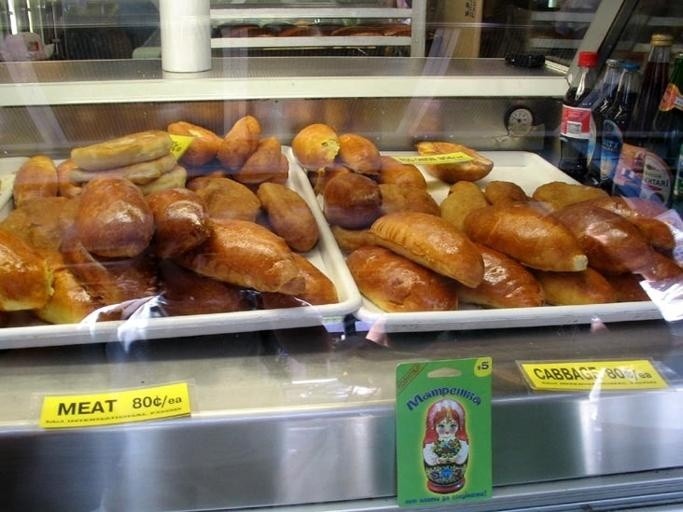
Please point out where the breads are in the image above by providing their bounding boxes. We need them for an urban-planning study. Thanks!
[291,114,682,313]
[0,115,338,326]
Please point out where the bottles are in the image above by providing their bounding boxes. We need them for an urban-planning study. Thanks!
[557,32,683,207]
